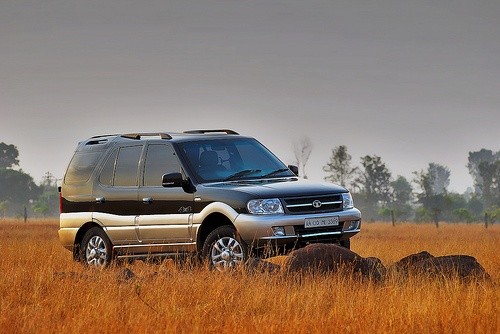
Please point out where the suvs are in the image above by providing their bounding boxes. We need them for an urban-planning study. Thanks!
[58,129,361,271]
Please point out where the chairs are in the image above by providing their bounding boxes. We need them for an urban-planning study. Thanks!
[199,151,231,179]
